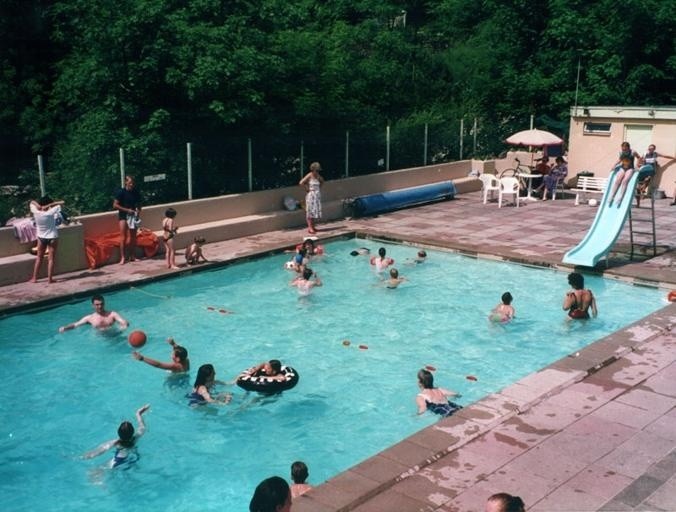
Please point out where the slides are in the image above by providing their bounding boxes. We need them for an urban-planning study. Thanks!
[562,165,654,268]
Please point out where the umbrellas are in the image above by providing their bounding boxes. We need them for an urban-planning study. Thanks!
[505,127,564,146]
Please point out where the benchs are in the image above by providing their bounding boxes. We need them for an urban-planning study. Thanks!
[570,176,609,206]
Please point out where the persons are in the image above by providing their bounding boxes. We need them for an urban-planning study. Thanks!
[527,141,676,201]
[487,493,526,512]
[162,208,180,269]
[132,335,189,392]
[30,195,65,283]
[490,292,515,322]
[416,369,464,418]
[113,175,141,265]
[249,476,293,512]
[59,295,129,335]
[290,462,312,499]
[230,359,284,419]
[185,236,208,264]
[185,364,239,417]
[284,239,426,303]
[299,161,324,233]
[562,272,597,327]
[80,403,150,471]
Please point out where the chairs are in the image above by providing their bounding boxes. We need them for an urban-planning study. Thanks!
[478,174,500,204]
[544,175,565,201]
[498,177,520,208]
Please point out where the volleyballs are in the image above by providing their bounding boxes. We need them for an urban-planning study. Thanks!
[129,331,146,347]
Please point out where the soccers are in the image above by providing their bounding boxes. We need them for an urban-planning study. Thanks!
[589,199,597,207]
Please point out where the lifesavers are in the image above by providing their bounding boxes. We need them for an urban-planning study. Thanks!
[296,244,324,255]
[370,256,393,266]
[236,365,298,392]
